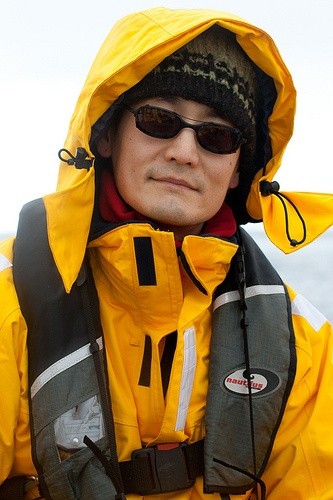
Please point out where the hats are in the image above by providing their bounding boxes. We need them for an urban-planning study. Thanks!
[124,26,261,165]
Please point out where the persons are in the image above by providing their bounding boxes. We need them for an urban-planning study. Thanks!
[0,7,333,500]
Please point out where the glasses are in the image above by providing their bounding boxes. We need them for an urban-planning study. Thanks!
[127,104,247,154]
